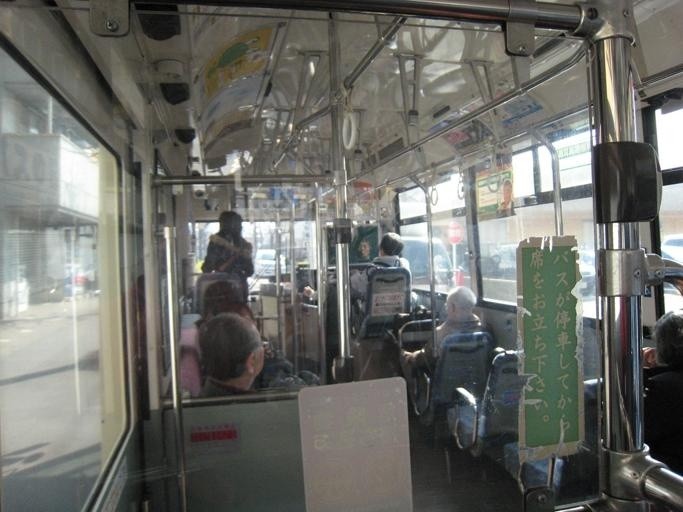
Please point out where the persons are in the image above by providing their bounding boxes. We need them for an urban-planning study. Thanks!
[195,311,265,399]
[303,285,318,306]
[203,211,254,303]
[399,286,483,380]
[209,303,256,328]
[642,310,683,474]
[202,280,242,316]
[352,238,374,263]
[500,181,512,209]
[350,231,411,321]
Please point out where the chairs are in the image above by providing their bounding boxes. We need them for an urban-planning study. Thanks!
[410,333,492,448]
[445,350,520,484]
[398,318,440,416]
[357,267,412,381]
[192,271,249,314]
[505,378,603,512]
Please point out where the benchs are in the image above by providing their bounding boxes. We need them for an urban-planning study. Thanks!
[177,311,203,397]
